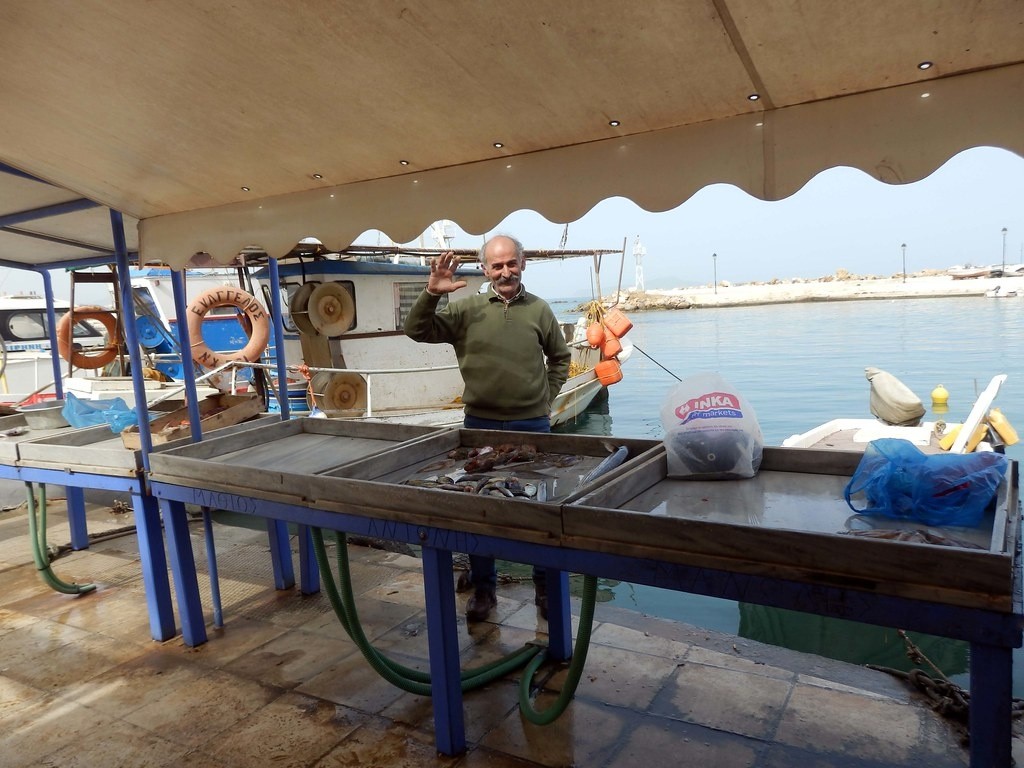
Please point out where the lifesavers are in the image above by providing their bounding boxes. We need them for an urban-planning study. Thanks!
[182,285,269,372]
[54,305,124,370]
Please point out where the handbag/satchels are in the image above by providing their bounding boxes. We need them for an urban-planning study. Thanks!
[657,370,763,477]
[844,438,1008,526]
[862,367,927,426]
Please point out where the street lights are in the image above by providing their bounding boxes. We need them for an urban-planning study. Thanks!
[901,243,907,283]
[711,253,720,295]
[1001,227,1008,275]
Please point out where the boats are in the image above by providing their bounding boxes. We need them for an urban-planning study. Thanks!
[777,366,1017,462]
[13,240,637,436]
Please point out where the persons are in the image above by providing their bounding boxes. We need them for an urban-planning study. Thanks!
[403,235,573,620]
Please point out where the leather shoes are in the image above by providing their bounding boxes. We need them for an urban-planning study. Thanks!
[466,586,498,619]
[534,590,550,622]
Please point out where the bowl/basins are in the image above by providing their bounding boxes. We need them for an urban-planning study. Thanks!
[15,398,90,430]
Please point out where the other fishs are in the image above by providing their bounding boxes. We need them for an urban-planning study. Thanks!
[399,474,536,500]
